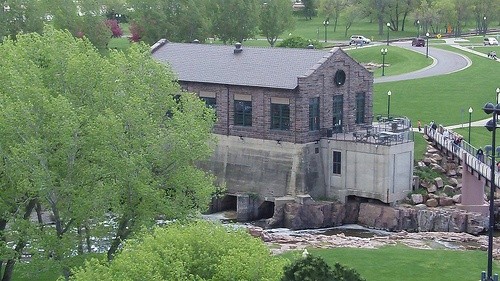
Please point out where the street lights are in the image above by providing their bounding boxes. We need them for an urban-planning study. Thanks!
[482,102,500,281]
[387,22,390,46]
[417,19,419,38]
[387,90,392,119]
[468,106,473,145]
[483,16,486,37]
[496,88,500,122]
[381,48,387,76]
[323,20,329,42]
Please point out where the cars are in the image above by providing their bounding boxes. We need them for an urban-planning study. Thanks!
[483,37,498,46]
[412,38,425,48]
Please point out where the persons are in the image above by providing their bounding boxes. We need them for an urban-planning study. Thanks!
[430,120,461,153]
[487,50,497,60]
[476,146,483,162]
[417,119,423,131]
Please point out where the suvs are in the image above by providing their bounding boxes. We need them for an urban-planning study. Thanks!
[425,32,430,58]
[350,35,371,44]
